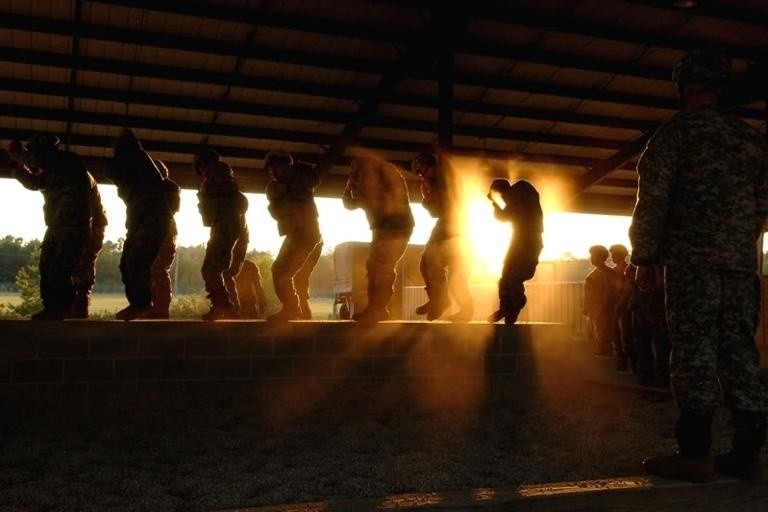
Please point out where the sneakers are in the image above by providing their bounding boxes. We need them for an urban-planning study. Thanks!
[488,292,529,325]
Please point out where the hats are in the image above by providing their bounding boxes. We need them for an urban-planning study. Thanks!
[112,127,143,155]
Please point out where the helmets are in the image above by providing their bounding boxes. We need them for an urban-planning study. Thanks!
[412,153,437,170]
[589,245,608,258]
[610,244,628,257]
[192,148,223,175]
[264,149,293,172]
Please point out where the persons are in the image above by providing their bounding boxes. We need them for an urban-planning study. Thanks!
[407,144,477,322]
[584,49,768,482]
[100,126,181,319]
[11,132,110,321]
[189,142,267,322]
[261,148,324,322]
[339,149,415,321]
[482,172,543,322]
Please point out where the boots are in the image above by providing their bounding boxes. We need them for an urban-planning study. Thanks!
[267,296,312,321]
[593,344,667,386]
[32,292,88,319]
[643,411,718,482]
[715,410,766,477]
[416,284,474,323]
[352,284,395,321]
[201,289,239,320]
[116,289,171,318]
